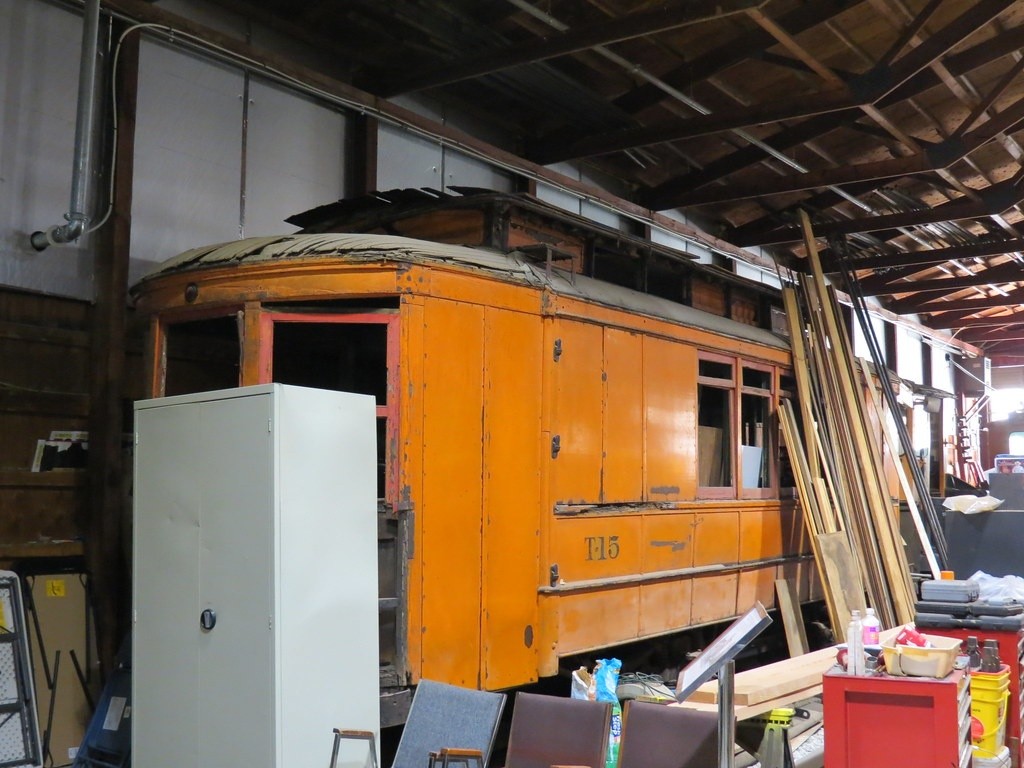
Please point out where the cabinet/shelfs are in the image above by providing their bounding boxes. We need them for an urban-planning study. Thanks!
[130,383,382,768]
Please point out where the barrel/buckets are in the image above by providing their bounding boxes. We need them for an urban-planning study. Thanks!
[969,664,1012,768]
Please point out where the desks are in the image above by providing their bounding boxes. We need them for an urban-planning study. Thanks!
[25,571,100,768]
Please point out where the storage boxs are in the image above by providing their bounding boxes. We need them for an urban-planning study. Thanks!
[880,634,963,678]
[389,203,788,335]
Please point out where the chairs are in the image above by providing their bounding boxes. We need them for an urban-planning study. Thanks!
[616,699,737,768]
[440,690,615,768]
[329,680,508,768]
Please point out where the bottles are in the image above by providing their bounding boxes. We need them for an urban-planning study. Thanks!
[862,608,880,646]
[847,610,865,676]
